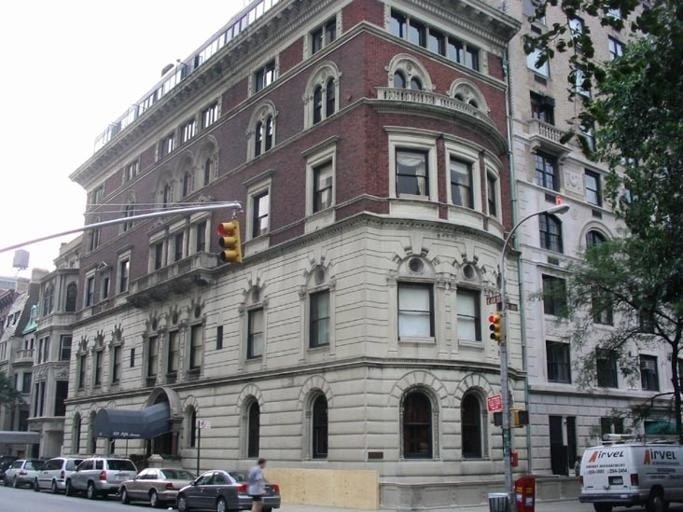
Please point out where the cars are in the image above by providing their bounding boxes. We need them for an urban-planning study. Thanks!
[0,453,283,512]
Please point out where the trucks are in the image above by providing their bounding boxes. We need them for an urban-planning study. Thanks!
[577,433,683,511]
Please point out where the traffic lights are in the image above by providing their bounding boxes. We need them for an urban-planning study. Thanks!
[487,313,503,342]
[215,218,245,268]
[508,407,528,428]
[492,410,503,426]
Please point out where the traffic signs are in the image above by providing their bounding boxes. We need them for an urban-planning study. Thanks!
[505,303,518,311]
[485,294,501,305]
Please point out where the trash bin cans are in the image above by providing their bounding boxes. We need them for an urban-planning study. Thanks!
[488,493,510,512]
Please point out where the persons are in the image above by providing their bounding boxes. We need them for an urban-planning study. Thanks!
[244,457,269,512]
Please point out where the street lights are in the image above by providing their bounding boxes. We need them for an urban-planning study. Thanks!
[496,203,570,511]
